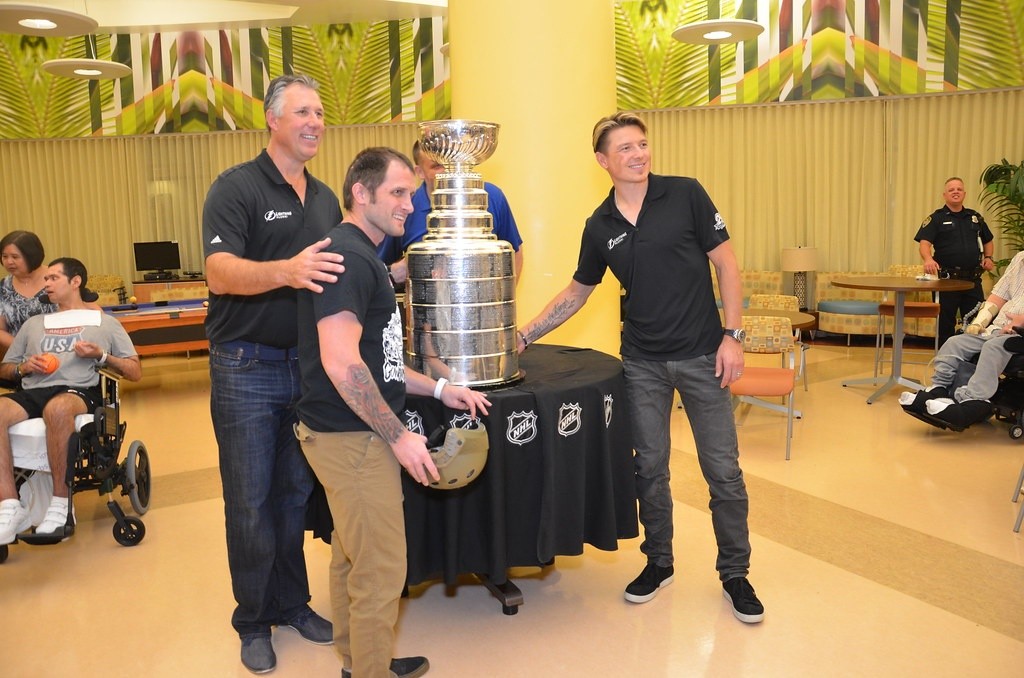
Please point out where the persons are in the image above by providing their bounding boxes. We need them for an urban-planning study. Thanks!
[294,146,493,678]
[0,258,143,545]
[899,250,1024,414]
[515,112,765,623]
[0,231,59,349]
[375,138,522,294]
[419,254,477,386]
[203,75,345,676]
[914,177,994,354]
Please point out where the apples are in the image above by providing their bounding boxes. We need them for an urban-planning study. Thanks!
[130,296,137,303]
[202,301,209,307]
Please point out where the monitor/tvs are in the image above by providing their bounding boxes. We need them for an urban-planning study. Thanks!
[134,241,181,275]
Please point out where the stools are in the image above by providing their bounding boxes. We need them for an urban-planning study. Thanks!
[819,300,880,314]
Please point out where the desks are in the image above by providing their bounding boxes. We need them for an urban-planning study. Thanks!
[676,309,816,407]
[130,280,209,302]
[303,342,639,615]
[100,296,209,359]
[830,276,975,404]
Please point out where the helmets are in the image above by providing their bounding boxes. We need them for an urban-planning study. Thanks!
[422,422,488,491]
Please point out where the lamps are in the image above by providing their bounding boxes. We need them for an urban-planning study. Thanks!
[671,18,764,46]
[782,245,817,311]
[0,0,132,83]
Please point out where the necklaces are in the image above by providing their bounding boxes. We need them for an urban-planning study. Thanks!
[22,281,31,286]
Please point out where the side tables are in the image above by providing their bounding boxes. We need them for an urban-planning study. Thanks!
[792,310,819,344]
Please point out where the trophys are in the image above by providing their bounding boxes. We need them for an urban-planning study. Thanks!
[405,120,526,389]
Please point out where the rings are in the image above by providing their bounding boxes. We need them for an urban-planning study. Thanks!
[737,373,741,376]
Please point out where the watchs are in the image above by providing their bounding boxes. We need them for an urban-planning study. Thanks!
[724,329,746,342]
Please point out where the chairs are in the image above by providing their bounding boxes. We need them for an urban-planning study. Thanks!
[874,263,942,389]
[728,295,810,461]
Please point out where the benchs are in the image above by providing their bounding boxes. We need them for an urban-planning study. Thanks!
[709,270,783,308]
[88,276,129,306]
[814,270,995,337]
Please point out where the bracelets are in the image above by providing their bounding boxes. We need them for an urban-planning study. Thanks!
[984,256,991,259]
[449,367,456,384]
[387,265,398,287]
[95,351,107,364]
[434,377,449,401]
[517,330,527,350]
[15,362,32,379]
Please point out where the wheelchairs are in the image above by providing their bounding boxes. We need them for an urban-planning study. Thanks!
[0,366,151,564]
[900,325,1024,440]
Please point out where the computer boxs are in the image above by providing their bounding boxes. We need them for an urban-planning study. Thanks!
[143,273,172,279]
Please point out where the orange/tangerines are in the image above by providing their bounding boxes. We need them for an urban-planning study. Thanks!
[38,354,59,374]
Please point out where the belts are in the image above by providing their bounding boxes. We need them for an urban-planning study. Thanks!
[210,339,298,361]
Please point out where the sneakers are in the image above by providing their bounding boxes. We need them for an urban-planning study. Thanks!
[624,563,673,604]
[0,499,32,545]
[722,576,764,623]
[240,638,276,673]
[36,496,77,535]
[341,656,430,678]
[270,607,334,645]
